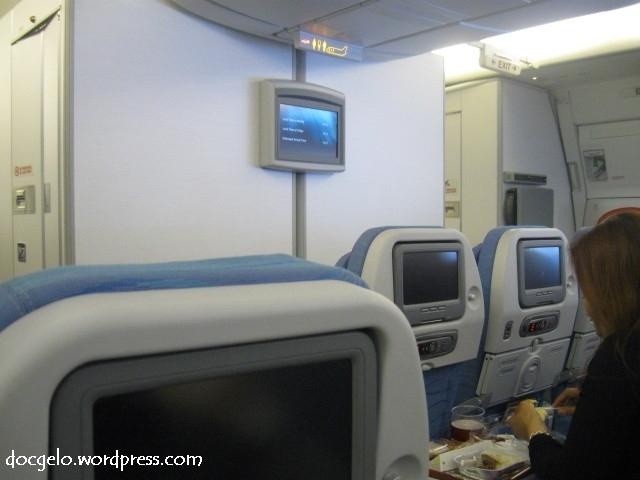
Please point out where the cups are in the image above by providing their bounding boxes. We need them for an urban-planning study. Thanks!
[450,405,487,442]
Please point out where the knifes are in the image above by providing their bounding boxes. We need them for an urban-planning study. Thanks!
[509,406,559,416]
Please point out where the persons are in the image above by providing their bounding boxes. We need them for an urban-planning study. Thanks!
[511,212,640,480]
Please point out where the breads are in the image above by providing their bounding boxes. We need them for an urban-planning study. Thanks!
[481,450,520,470]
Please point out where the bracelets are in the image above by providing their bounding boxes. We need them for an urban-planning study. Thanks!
[528,431,550,443]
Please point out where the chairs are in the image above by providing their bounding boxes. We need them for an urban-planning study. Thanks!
[0,223,606,480]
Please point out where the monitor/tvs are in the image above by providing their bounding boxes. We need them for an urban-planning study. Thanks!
[518,239,566,307]
[260,78,347,172]
[50,330,378,480]
[393,242,466,327]
